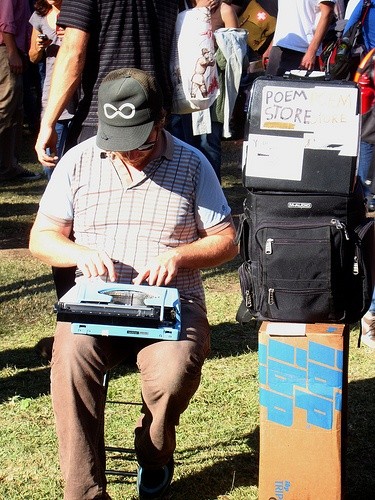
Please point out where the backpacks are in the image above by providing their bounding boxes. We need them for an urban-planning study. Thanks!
[235,175,374,325]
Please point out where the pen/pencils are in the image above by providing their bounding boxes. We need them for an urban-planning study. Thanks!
[75,258,120,277]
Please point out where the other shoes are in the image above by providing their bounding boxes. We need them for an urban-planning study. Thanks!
[361,311,375,348]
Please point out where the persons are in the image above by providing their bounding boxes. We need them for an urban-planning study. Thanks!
[29,70,239,500]
[0,0,375,357]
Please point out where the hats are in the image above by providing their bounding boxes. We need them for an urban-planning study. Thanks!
[96,68,162,152]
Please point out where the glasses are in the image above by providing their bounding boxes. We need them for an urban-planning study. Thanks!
[134,127,159,151]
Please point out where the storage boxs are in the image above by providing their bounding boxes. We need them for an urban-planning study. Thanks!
[257,321,349,500]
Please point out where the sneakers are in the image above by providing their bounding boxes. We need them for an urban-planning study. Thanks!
[137,455,174,500]
[19,169,41,180]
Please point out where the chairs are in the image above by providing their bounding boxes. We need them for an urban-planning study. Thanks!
[51,233,143,479]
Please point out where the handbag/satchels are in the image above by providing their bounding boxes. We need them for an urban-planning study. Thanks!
[320,0,371,80]
[239,0,277,51]
[170,0,220,114]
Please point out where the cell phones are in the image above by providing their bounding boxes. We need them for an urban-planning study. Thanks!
[38,34,48,40]
